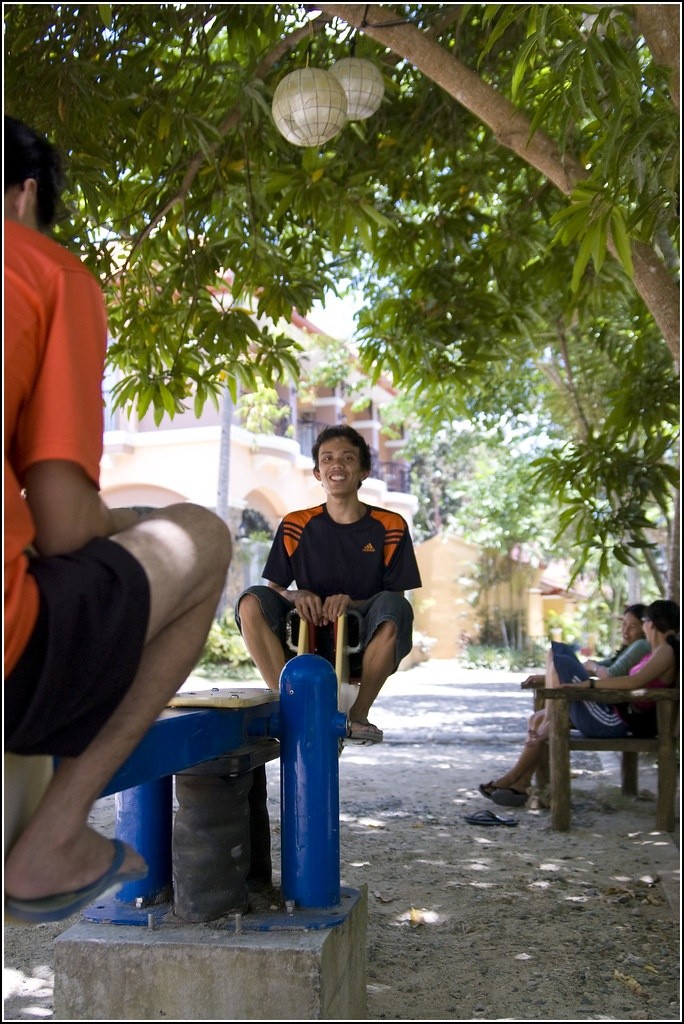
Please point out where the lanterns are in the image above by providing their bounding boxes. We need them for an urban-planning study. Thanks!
[328,58,384,121]
[272,66,348,147]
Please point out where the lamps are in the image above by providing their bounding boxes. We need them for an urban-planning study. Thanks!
[271,14,347,147]
[328,36,386,122]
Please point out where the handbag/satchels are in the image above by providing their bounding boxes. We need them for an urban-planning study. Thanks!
[616,702,658,738]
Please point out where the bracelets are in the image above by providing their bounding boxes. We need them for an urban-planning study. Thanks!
[594,666,599,672]
[590,679,594,688]
[132,506,153,517]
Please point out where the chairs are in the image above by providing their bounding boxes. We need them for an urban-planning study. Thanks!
[520,678,679,830]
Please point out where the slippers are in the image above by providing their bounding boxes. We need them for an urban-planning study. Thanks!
[464,810,520,827]
[479,781,529,807]
[4,839,148,922]
[349,720,383,739]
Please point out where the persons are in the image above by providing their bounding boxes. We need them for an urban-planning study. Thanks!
[522,604,649,742]
[235,425,422,743]
[5,113,232,924]
[479,598,680,808]
